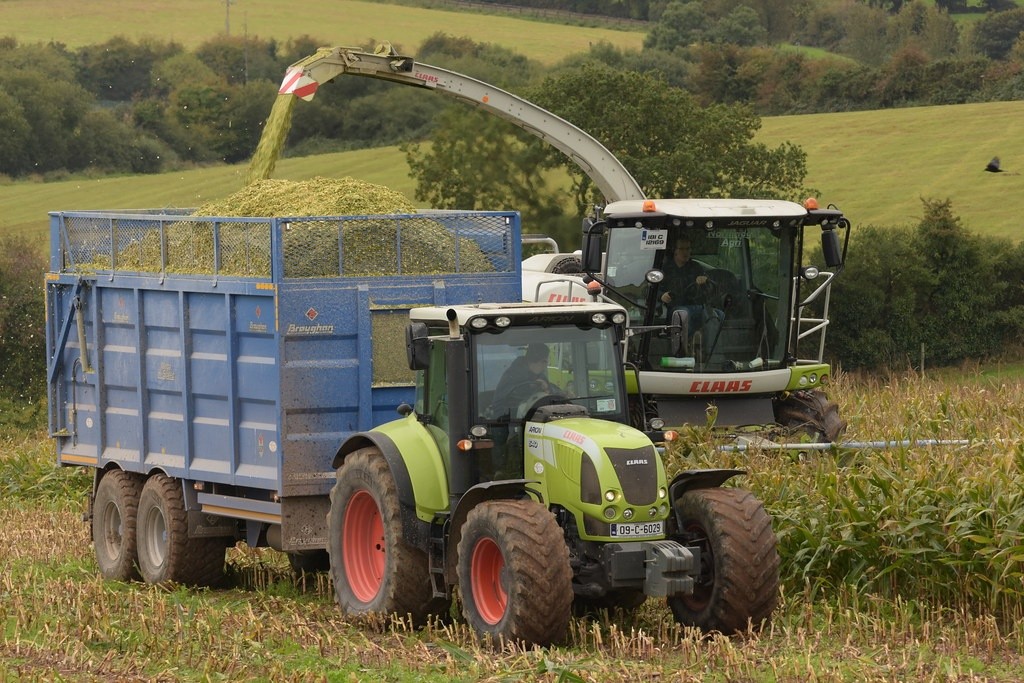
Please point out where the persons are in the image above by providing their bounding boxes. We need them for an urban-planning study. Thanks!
[641,235,709,315]
[493,343,550,410]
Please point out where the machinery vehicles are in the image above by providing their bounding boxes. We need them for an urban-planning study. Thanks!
[243,42,854,458]
[42,209,781,644]
